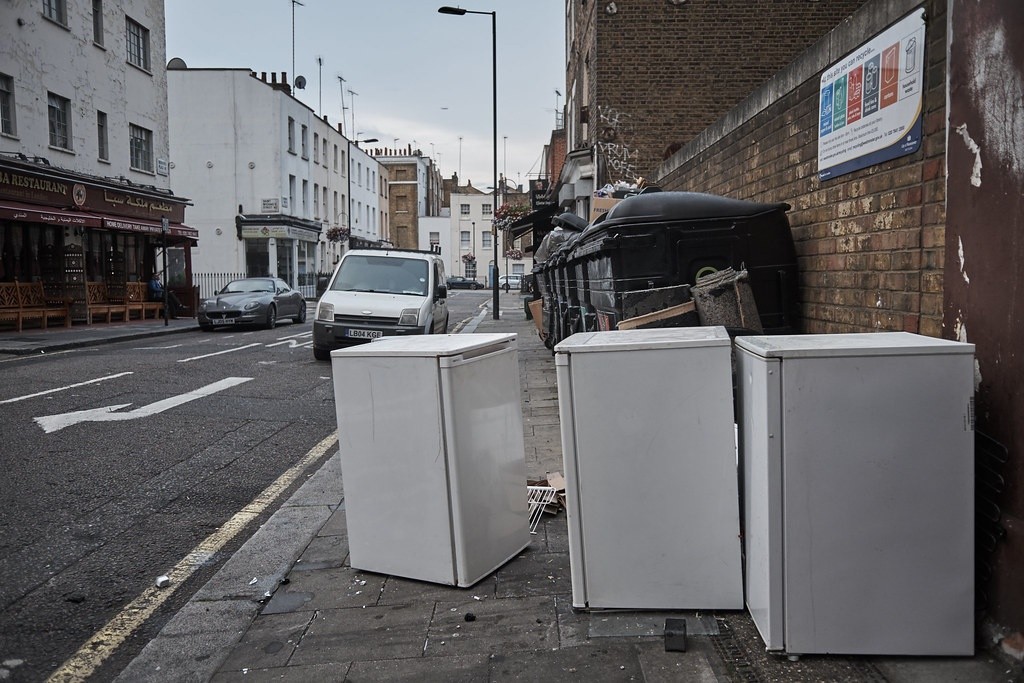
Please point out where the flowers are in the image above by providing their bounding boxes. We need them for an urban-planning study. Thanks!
[326,225,350,244]
[505,248,526,260]
[493,200,531,231]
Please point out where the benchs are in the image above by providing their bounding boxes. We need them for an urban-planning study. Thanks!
[1,281,166,337]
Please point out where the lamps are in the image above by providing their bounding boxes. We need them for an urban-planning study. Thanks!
[26,156,50,166]
[146,186,174,196]
[105,176,132,186]
[125,183,156,192]
[0,150,28,161]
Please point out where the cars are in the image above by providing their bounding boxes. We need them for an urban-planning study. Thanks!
[446,276,484,290]
[198,278,306,332]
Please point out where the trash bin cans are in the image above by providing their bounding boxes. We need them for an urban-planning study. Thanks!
[529,190,802,356]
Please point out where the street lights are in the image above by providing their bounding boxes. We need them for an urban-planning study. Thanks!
[347,138,378,249]
[437,5,500,319]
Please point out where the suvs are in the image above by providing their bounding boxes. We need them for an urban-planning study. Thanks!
[499,275,524,290]
[310,236,451,361]
